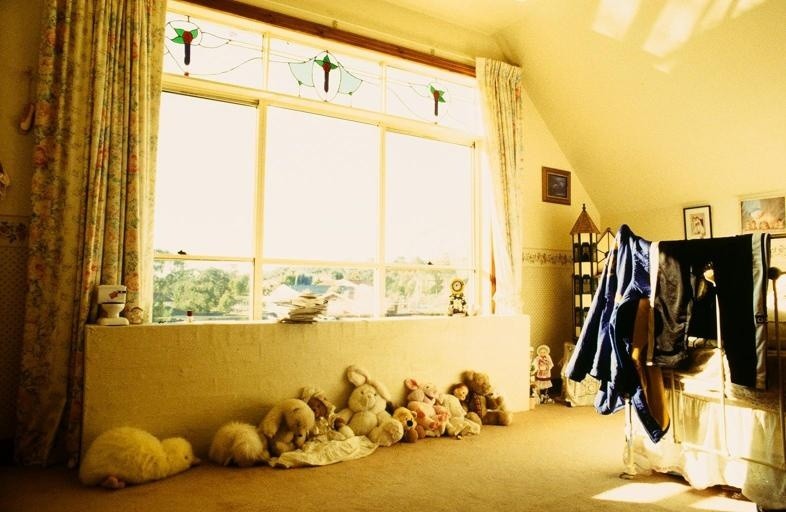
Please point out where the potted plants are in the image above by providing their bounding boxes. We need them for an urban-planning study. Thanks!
[736,193,786,235]
[683,205,713,240]
[542,166,571,206]
